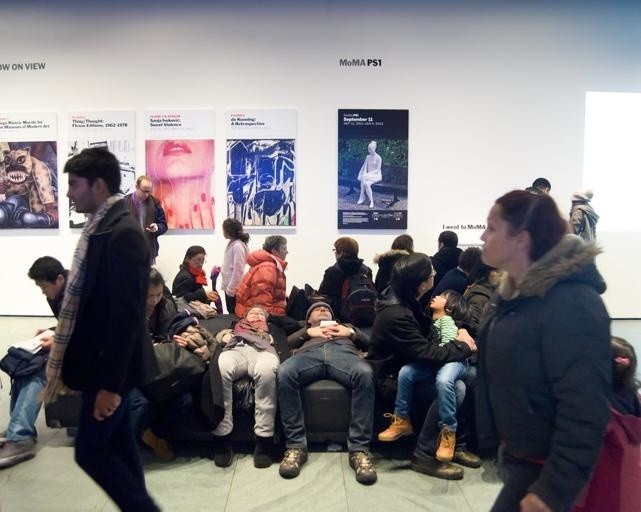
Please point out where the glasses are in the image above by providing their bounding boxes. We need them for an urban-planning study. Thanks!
[429,269,436,277]
[138,186,149,193]
[510,187,543,235]
[332,249,336,253]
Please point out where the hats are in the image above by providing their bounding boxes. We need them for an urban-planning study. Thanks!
[305,302,333,325]
[571,190,593,202]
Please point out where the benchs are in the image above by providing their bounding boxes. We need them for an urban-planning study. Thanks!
[44,314,474,448]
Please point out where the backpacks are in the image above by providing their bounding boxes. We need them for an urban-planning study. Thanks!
[334,263,378,329]
[568,407,641,512]
[0,347,48,395]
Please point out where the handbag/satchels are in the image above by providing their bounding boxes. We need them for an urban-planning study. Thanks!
[147,336,206,392]
[304,284,331,306]
[287,286,307,320]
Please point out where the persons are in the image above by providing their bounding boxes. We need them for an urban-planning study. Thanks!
[479,189,610,511]
[122,176,168,265]
[145,141,214,232]
[356,140,382,208]
[525,178,551,196]
[569,189,601,244]
[62,146,162,512]
[136,219,501,485]
[0,256,70,468]
[570,336,641,512]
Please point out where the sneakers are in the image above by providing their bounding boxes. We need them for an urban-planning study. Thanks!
[0,438,35,466]
[278,448,306,477]
[141,427,175,460]
[376,413,413,442]
[0,425,37,442]
[211,434,233,466]
[348,449,376,485]
[411,426,482,480]
[253,436,273,468]
